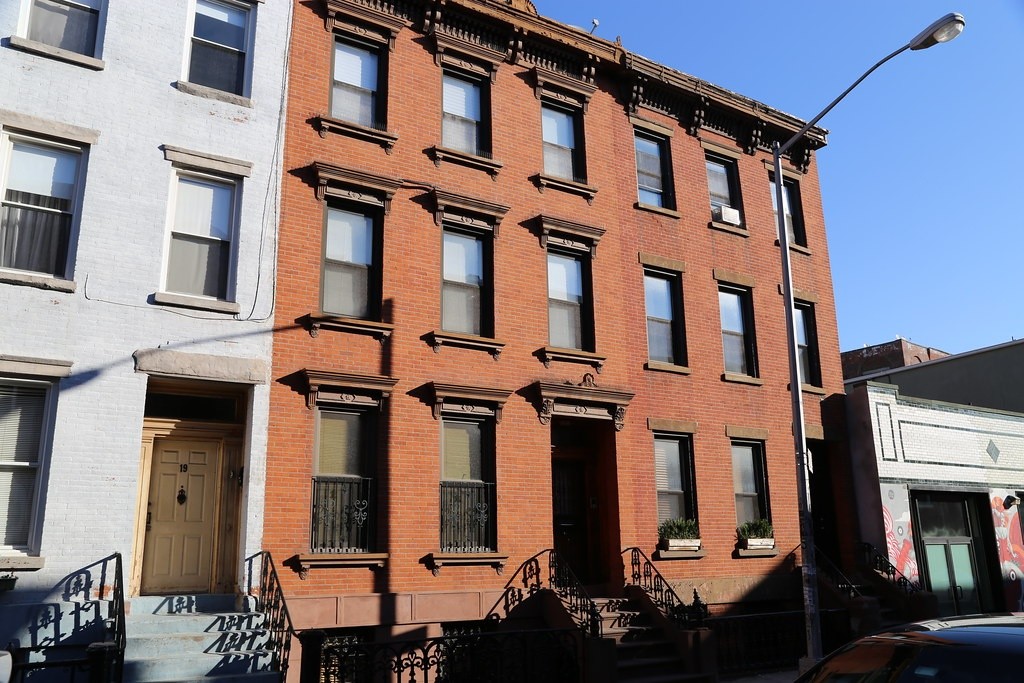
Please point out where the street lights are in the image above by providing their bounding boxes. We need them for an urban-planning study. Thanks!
[773,13,967,670]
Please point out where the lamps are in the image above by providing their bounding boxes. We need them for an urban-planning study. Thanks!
[1005,494,1021,506]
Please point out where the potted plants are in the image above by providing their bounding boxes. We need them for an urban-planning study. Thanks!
[736,518,775,550]
[0,572,19,590]
[658,515,701,550]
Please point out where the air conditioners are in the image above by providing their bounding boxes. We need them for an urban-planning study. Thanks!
[714,206,740,227]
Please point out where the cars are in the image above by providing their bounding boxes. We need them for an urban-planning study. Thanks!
[793,613,1024,683]
